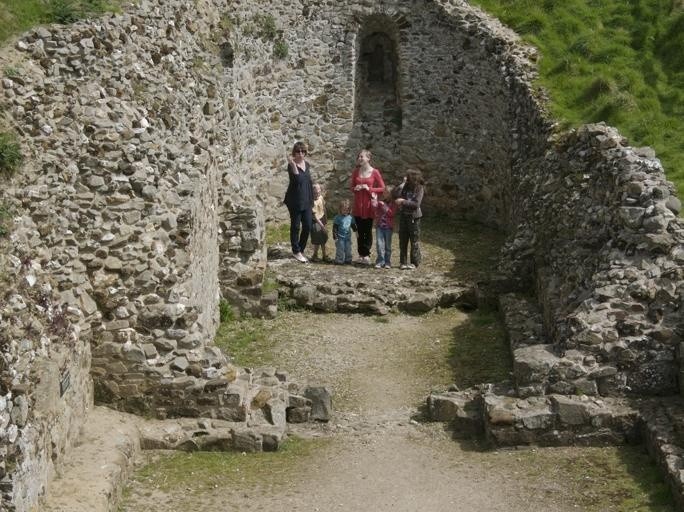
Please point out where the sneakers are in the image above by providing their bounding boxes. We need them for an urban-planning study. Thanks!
[291,252,418,269]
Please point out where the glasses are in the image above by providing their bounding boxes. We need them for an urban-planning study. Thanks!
[295,150,306,154]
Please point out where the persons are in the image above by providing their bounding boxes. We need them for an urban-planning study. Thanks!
[349,148,386,264]
[392,167,424,269]
[330,199,359,265]
[281,141,314,264]
[309,182,333,263]
[369,184,398,270]
